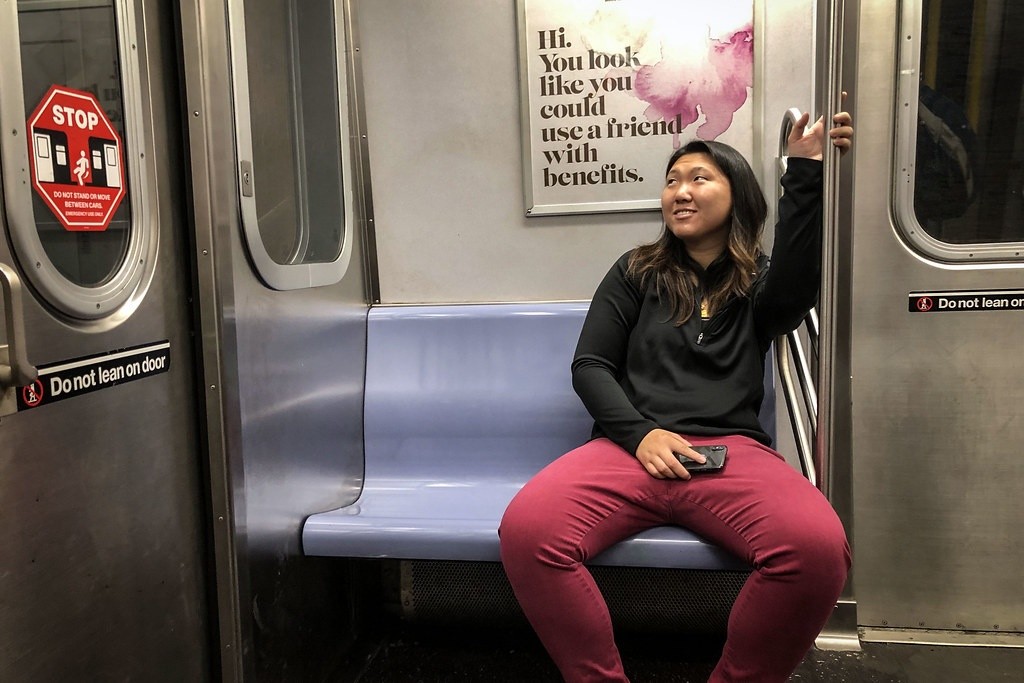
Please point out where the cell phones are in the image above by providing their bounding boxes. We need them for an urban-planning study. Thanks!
[671,445,729,471]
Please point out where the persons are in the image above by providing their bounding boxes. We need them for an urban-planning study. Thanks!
[499,91,856,683]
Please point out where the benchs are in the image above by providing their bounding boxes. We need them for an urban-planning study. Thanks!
[301,296,784,636]
